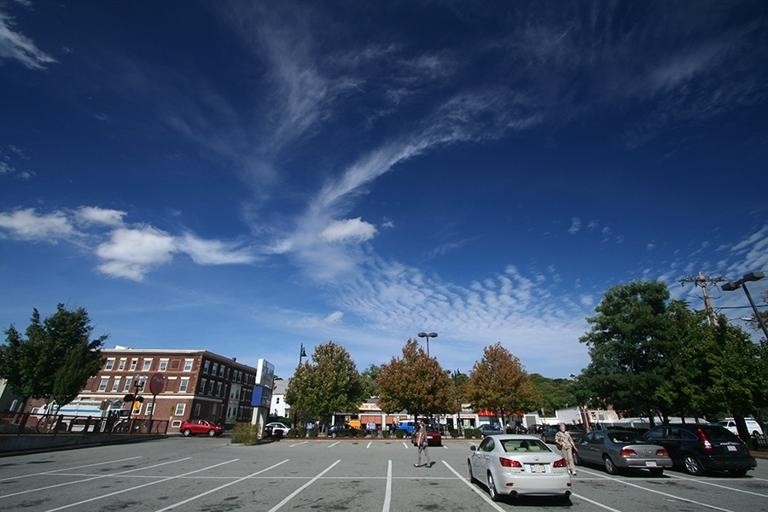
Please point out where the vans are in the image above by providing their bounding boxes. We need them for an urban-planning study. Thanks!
[721,420,764,438]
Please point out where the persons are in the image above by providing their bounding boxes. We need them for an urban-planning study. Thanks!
[413,421,432,469]
[554,422,580,476]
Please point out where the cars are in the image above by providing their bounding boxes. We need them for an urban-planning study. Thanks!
[642,423,757,476]
[328,424,366,438]
[572,428,674,477]
[264,421,292,437]
[470,423,551,434]
[180,417,224,437]
[467,433,572,502]
[393,421,418,439]
[411,424,441,446]
[542,424,586,443]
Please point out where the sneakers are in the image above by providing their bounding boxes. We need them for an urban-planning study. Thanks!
[414,463,431,468]
[568,470,577,475]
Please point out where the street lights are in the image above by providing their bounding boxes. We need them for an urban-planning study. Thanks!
[721,271,768,339]
[418,331,438,359]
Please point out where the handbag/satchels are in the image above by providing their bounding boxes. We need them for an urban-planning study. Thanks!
[414,437,424,447]
[556,442,562,450]
